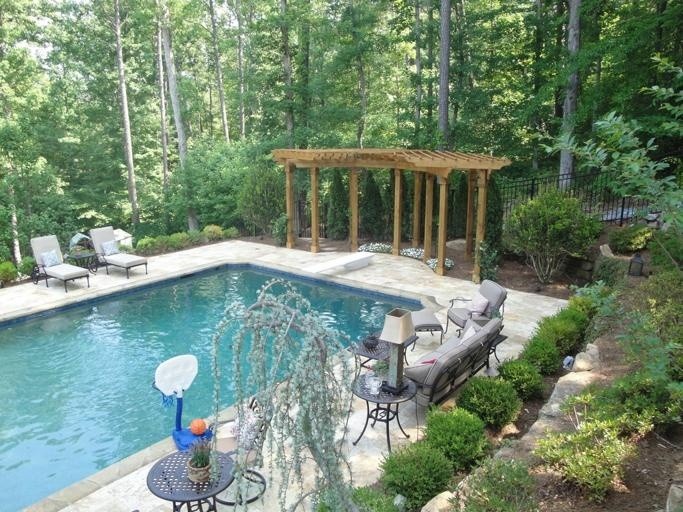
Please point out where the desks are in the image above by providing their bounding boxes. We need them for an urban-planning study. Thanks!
[488,335,508,365]
[348,369,419,459]
[144,447,240,512]
[66,252,97,276]
[347,330,420,377]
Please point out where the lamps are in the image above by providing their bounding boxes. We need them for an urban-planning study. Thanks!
[370,306,423,397]
[625,248,646,279]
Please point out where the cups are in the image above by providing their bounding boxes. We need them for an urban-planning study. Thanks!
[363,371,381,396]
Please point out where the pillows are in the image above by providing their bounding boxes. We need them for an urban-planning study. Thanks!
[37,248,64,271]
[100,239,123,259]
[456,324,477,346]
[464,290,491,318]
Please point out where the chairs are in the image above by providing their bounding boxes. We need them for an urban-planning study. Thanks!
[28,234,91,294]
[89,225,148,282]
[205,391,276,506]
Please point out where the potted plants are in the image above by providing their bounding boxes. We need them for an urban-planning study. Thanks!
[186,436,217,488]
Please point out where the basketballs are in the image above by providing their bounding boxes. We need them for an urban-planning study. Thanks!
[191,419,206,435]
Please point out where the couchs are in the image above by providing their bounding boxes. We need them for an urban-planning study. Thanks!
[409,308,446,353]
[441,278,509,339]
[406,315,509,416]
